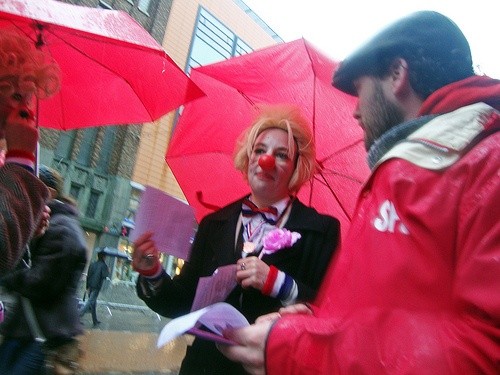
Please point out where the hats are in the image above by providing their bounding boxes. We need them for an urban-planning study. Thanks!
[331,10,472,96]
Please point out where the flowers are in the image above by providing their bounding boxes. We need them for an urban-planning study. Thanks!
[260,228,302,255]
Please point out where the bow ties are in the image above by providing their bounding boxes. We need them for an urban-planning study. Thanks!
[241,199,278,225]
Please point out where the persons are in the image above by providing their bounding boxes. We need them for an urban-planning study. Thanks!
[0,30,51,374]
[77,250,111,327]
[131,100,342,375]
[0,164,91,375]
[214,9,499,375]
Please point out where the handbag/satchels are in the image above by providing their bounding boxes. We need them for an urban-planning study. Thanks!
[39,334,86,369]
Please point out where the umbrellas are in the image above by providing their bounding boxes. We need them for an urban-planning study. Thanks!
[164,35,379,237]
[95,245,129,259]
[0,0,209,131]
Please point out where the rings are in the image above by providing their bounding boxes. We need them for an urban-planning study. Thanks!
[18,109,29,119]
[241,263,246,271]
[145,254,157,261]
